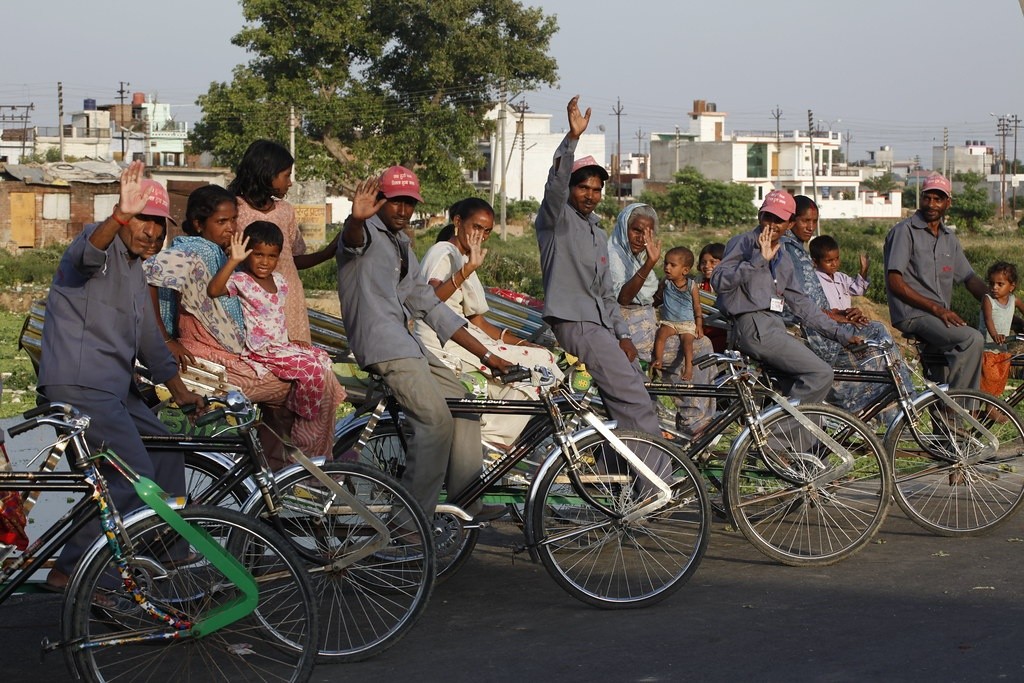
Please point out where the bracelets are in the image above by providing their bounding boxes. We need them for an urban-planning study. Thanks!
[451,273,459,289]
[462,265,469,280]
[500,327,508,340]
[480,351,493,365]
[113,211,129,226]
[515,339,526,346]
[165,338,171,343]
[636,272,646,280]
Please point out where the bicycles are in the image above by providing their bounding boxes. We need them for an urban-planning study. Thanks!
[0,335,1024,683]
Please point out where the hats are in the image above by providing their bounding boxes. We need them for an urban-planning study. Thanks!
[140,179,178,227]
[378,167,424,202]
[921,175,951,198]
[760,190,796,221]
[571,155,609,181]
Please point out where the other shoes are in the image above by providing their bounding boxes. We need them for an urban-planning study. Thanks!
[462,504,513,525]
[385,517,424,550]
[671,475,694,493]
[692,419,732,433]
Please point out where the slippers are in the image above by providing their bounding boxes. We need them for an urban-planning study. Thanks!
[763,446,792,469]
[933,410,965,436]
[154,553,204,570]
[950,467,1000,486]
[39,581,115,607]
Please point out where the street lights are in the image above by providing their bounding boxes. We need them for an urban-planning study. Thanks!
[990,112,1011,159]
[818,119,842,131]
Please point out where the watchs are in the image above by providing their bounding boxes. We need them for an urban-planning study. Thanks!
[621,334,632,340]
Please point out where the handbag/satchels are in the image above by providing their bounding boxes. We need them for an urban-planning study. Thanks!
[0,444,29,550]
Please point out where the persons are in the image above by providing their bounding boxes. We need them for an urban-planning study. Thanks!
[882,175,1024,487]
[977,261,1024,423]
[693,243,728,359]
[607,203,734,435]
[782,195,917,425]
[652,246,704,380]
[336,166,513,547]
[207,220,331,437]
[535,95,672,500]
[227,139,341,344]
[37,159,210,608]
[142,184,347,486]
[413,197,566,445]
[710,190,863,467]
[809,235,870,311]
